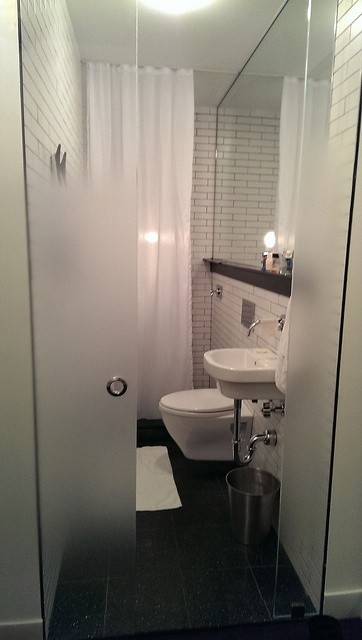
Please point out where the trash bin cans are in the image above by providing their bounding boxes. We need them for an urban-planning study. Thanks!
[225,468,280,546]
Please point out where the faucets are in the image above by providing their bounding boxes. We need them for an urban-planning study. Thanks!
[248,319,287,337]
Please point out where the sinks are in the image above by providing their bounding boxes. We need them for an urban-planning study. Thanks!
[203,347,279,402]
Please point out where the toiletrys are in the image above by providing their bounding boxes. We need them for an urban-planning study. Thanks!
[284,250,293,274]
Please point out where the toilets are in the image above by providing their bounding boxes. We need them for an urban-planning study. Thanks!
[158,389,252,461]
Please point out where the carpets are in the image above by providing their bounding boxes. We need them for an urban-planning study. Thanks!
[137,446,181,510]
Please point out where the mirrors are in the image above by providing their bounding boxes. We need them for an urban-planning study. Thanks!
[211,0,338,274]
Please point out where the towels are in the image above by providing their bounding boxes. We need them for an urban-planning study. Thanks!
[274,294,292,393]
